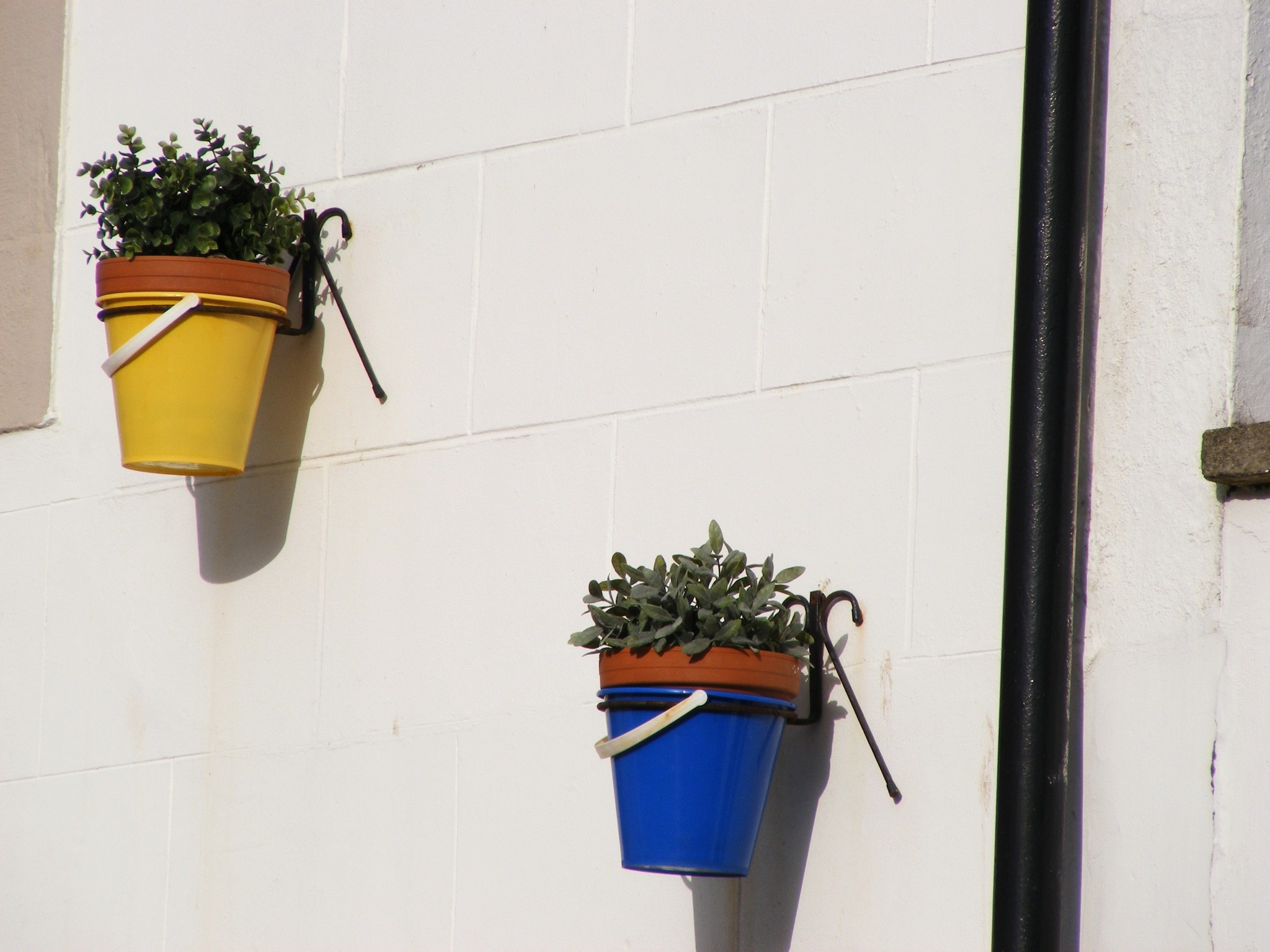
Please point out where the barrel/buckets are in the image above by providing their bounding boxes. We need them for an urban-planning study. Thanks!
[593,687,798,875]
[96,290,290,478]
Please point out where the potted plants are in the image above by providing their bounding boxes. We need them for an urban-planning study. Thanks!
[565,530,817,695]
[75,112,308,296]
